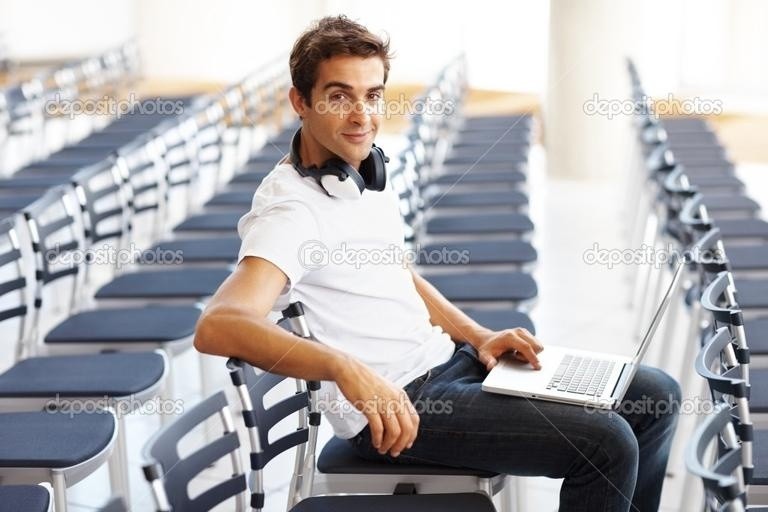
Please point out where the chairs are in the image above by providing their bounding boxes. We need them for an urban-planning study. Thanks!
[140,388,247,512]
[0,410,126,512]
[0,213,167,512]
[24,180,216,469]
[282,300,511,512]
[225,316,497,512]
[0,481,55,512]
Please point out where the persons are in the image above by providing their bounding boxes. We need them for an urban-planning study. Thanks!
[193,16,681,512]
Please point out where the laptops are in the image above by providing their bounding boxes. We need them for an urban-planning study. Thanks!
[481,257,684,411]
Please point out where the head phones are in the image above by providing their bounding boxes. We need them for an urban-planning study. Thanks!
[290,126,390,202]
